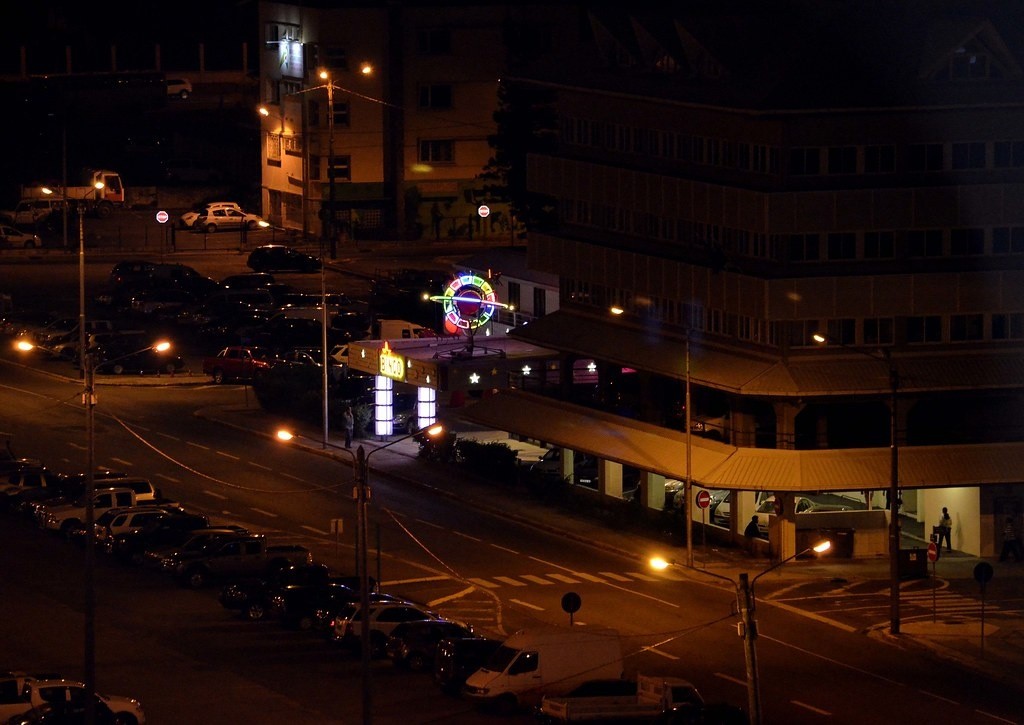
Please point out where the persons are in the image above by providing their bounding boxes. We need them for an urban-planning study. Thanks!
[861,490,873,510]
[343,407,354,449]
[744,515,768,540]
[938,507,952,553]
[883,490,901,510]
[997,517,1021,563]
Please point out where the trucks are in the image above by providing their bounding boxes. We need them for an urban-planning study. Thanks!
[20,169,125,220]
[540,672,706,725]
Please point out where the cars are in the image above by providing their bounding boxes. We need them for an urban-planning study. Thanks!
[196,206,261,233]
[0,257,1022,725]
[685,704,750,725]
[0,225,42,250]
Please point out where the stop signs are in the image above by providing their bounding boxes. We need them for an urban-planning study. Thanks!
[156,210,169,223]
[696,490,710,508]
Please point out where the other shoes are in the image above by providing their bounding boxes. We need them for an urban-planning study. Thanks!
[345,446,352,449]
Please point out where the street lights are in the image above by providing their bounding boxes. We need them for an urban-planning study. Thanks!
[252,65,396,257]
[649,538,831,725]
[262,263,329,450]
[607,304,692,569]
[811,332,899,635]
[38,178,105,434]
[273,423,444,725]
[10,335,175,725]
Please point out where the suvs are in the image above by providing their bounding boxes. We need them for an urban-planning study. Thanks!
[247,245,323,274]
[0,198,73,235]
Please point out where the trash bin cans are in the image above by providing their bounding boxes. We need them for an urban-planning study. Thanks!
[899,548,928,577]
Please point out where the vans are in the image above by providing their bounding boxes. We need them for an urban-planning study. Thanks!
[464,622,627,717]
[194,201,241,218]
